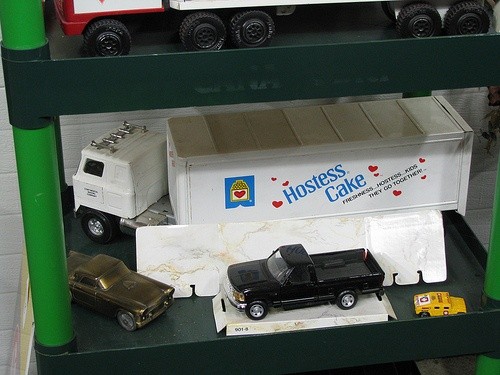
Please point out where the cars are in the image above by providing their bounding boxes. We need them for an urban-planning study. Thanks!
[66,249,175,332]
[413,291,468,319]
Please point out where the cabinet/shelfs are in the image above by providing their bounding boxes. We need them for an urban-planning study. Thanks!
[0,0,500,375]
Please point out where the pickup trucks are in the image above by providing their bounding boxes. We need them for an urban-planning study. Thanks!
[224,243,386,322]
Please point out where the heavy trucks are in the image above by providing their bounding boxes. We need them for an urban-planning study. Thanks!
[72,94,475,246]
[52,0,495,61]
